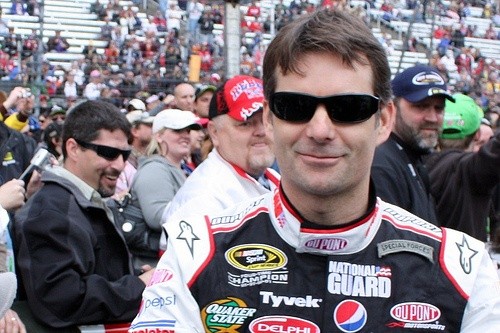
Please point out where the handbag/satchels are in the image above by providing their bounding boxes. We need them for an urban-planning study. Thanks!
[107,159,177,257]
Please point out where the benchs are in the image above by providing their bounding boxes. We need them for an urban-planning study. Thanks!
[0,0,500,91]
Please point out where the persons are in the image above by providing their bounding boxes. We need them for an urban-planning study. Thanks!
[129,7,500,333]
[0,0,500,280]
[160,77,281,257]
[373,66,455,225]
[12,100,155,333]
[0,206,26,333]
[425,93,500,243]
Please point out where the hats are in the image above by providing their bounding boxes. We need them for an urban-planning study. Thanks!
[152,110,201,132]
[130,99,147,111]
[391,64,457,103]
[441,92,483,138]
[127,111,155,123]
[48,104,65,116]
[90,70,101,77]
[208,75,264,121]
[195,84,217,97]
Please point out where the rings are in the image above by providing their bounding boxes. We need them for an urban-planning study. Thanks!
[11,317,16,320]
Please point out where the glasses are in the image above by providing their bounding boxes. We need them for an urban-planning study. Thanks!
[75,139,132,162]
[52,117,66,121]
[269,92,385,123]
[39,120,44,123]
